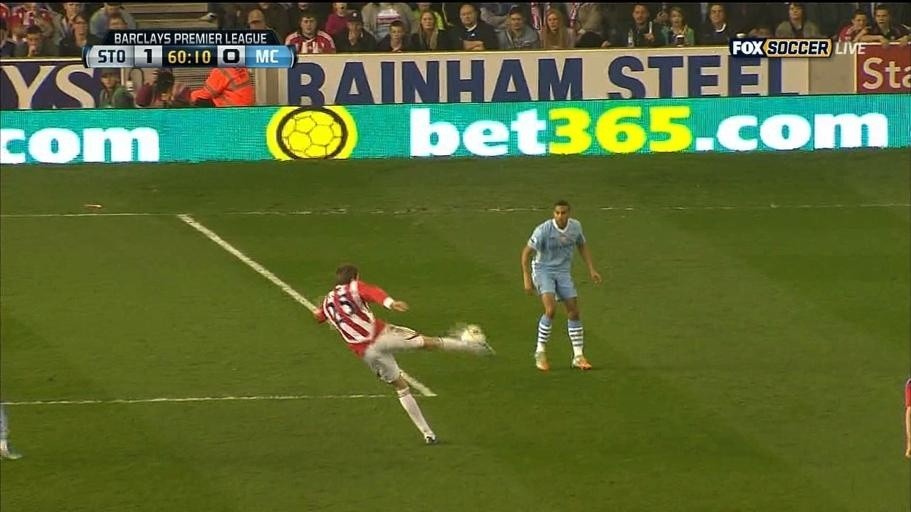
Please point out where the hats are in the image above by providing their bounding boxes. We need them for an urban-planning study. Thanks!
[346,10,363,23]
[246,9,266,26]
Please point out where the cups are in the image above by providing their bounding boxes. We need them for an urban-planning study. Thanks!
[677,34,686,45]
[736,33,748,38]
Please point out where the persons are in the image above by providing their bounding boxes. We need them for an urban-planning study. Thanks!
[1,2,910,110]
[520,199,602,371]
[312,265,496,446]
[905,377,911,459]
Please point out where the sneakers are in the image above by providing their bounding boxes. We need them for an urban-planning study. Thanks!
[0,448,25,463]
[461,328,497,358]
[424,432,439,444]
[533,350,550,370]
[569,357,594,370]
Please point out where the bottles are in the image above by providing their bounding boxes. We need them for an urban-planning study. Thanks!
[628,27,635,48]
